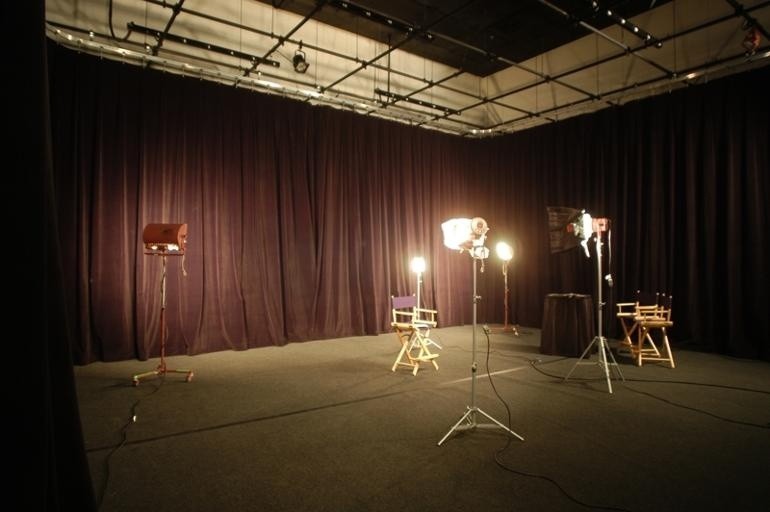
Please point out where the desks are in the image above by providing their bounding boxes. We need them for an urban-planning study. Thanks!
[538,293,598,359]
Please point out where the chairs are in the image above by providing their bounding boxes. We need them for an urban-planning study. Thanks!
[390,294,439,375]
[612,289,676,369]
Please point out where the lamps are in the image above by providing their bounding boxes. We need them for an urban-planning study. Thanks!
[741,21,762,50]
[126,222,195,257]
[292,41,309,73]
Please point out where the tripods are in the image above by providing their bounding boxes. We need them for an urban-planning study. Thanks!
[437,253,525,446]
[561,226,625,394]
